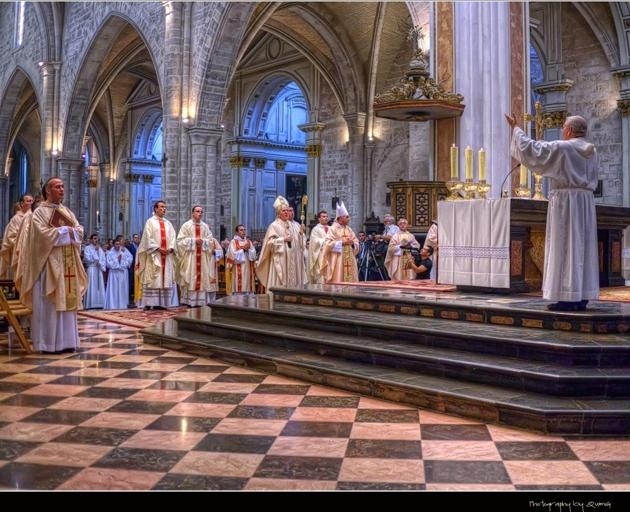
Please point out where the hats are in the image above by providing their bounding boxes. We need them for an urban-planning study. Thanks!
[384,216,393,224]
[273,196,289,210]
[336,201,349,221]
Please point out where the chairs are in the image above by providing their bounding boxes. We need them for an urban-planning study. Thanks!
[0,284,34,354]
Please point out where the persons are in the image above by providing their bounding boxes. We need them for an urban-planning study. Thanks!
[1,176,437,355]
[505,111,599,312]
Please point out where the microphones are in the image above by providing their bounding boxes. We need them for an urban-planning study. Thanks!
[501,164,521,197]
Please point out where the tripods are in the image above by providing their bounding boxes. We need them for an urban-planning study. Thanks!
[358,240,385,282]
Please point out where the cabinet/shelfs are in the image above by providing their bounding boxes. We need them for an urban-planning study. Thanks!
[387,180,413,229]
[411,181,436,230]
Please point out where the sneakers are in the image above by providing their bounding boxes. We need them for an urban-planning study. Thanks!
[548,304,577,311]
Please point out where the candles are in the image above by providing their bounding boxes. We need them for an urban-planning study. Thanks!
[95,209,100,231]
[464,145,474,181]
[478,147,487,181]
[449,143,460,181]
[520,163,528,186]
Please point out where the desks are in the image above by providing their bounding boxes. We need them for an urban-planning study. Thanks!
[437,197,629,298]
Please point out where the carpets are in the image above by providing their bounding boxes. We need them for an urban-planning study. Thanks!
[524,284,630,304]
[77,303,198,328]
[326,278,456,292]
